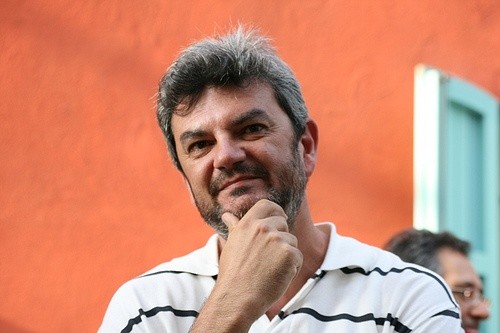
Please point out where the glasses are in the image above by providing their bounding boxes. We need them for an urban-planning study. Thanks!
[452,287,492,306]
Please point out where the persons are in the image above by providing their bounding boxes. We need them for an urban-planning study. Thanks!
[384,229,489,333]
[99,28,465,333]
